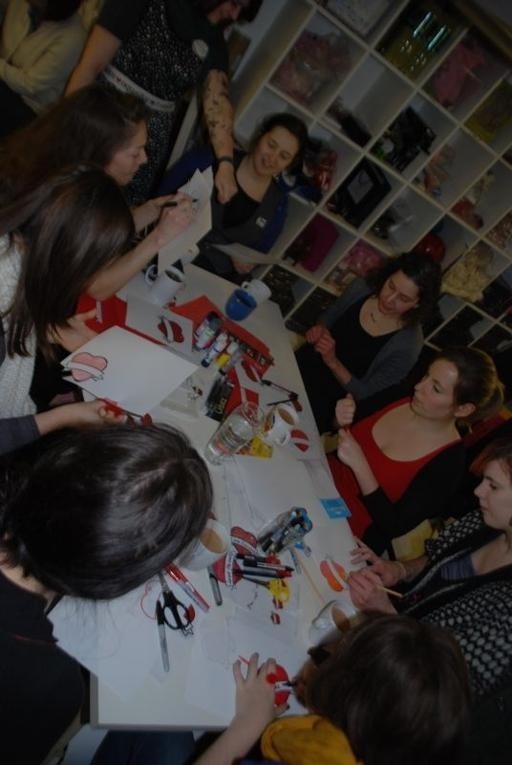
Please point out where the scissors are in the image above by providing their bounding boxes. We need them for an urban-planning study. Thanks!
[158,571,190,631]
[94,397,141,418]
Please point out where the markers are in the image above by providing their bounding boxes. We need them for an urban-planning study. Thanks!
[264,379,298,399]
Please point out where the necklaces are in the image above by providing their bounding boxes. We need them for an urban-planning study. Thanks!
[367,294,408,330]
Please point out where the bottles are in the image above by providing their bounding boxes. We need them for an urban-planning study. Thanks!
[403,25,452,78]
[384,11,435,69]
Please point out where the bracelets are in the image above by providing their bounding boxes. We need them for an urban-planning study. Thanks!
[217,155,237,168]
[395,558,418,584]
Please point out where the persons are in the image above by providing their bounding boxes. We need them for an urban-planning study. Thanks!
[325,346,505,541]
[1,166,137,454]
[1,81,195,302]
[0,1,103,136]
[152,110,309,282]
[0,422,215,762]
[65,2,265,206]
[346,441,510,711]
[192,614,471,765]
[294,250,445,437]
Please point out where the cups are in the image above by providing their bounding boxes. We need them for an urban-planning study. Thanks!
[144,264,186,301]
[263,405,297,450]
[205,401,260,467]
[178,519,231,571]
[225,290,257,322]
[241,279,271,305]
[307,601,355,643]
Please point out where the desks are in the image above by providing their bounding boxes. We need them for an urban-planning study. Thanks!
[46,256,375,765]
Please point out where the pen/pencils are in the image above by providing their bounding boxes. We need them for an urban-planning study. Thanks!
[207,564,224,605]
[372,581,402,599]
[235,554,296,587]
[159,198,199,209]
[259,506,308,553]
[156,600,170,673]
[267,397,294,406]
[165,561,209,611]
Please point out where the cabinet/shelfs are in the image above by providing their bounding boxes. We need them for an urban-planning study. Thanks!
[205,1,512,368]
